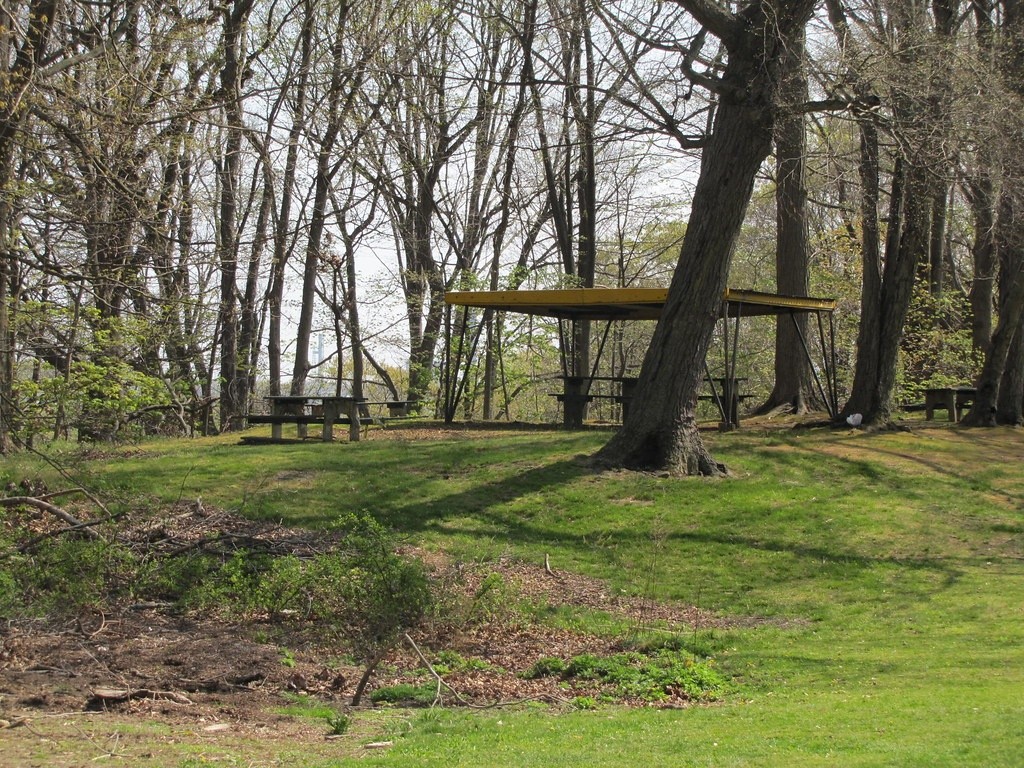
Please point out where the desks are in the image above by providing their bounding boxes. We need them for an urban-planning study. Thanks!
[566,375,748,428]
[385,401,411,417]
[263,394,368,444]
[919,386,976,421]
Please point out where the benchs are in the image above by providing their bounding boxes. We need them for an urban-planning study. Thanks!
[248,414,323,426]
[899,403,947,411]
[314,418,372,425]
[549,391,754,403]
